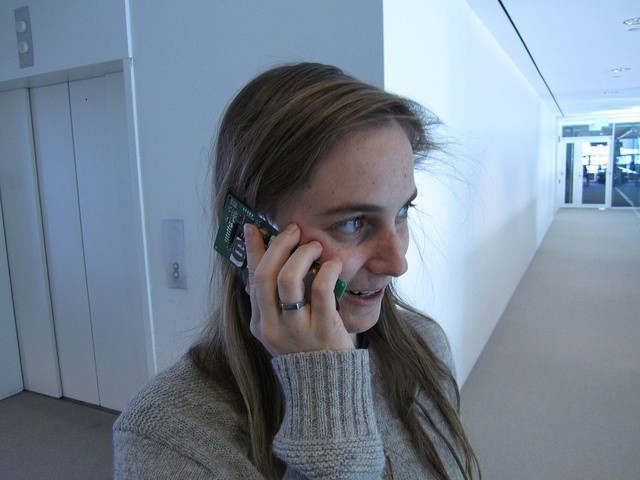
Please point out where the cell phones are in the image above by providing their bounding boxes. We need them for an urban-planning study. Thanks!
[211,193,348,313]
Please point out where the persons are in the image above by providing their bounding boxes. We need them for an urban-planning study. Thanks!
[112,63,481,479]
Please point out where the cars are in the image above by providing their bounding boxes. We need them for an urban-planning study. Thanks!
[597,166,637,183]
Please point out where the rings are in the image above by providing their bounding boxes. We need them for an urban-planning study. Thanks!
[277,297,309,310]
